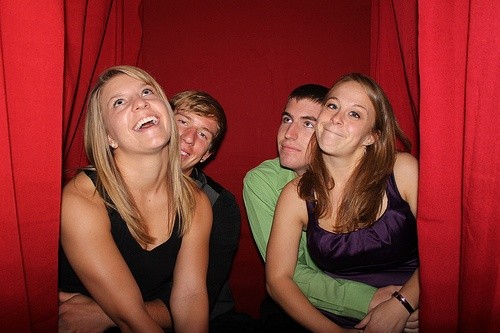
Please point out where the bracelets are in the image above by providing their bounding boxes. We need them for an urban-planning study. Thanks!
[391,291,415,315]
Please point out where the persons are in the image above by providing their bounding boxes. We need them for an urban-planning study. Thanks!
[265,72,418,333]
[58,89,241,333]
[238,83,418,333]
[58,64,214,333]
